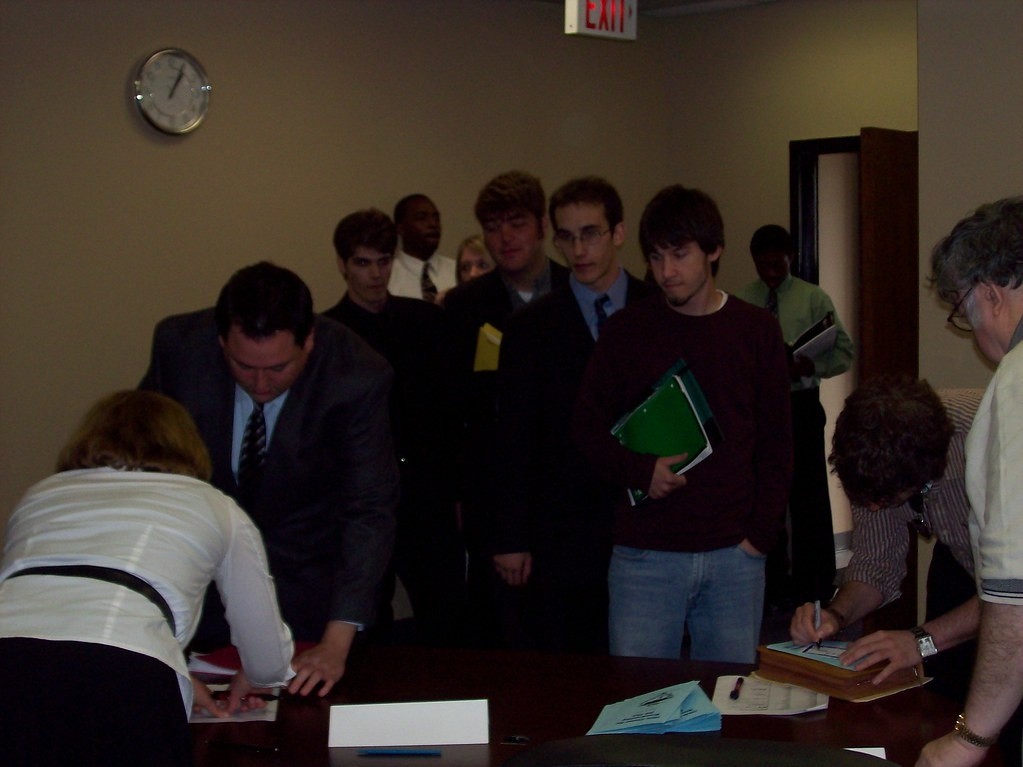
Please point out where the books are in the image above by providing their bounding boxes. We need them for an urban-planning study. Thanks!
[611,375,713,506]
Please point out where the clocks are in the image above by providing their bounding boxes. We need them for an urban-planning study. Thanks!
[132,46,214,138]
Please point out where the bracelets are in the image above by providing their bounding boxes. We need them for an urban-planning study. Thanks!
[827,607,846,630]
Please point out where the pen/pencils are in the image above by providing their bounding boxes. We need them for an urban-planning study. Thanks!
[814,600,821,649]
[357,749,442,756]
[731,678,743,699]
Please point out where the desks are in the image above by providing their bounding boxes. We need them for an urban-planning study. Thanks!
[186,646,999,767]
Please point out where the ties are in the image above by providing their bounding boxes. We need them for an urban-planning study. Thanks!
[765,289,776,317]
[422,264,438,304]
[594,294,610,336]
[237,401,266,503]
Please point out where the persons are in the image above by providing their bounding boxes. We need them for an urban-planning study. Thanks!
[0,393,296,767]
[137,261,401,697]
[733,223,853,611]
[790,376,986,705]
[914,195,1023,767]
[317,168,789,664]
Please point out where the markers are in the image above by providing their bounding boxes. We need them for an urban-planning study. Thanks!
[211,691,280,701]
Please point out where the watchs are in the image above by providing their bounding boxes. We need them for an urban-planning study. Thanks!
[911,625,939,662]
[955,712,1000,748]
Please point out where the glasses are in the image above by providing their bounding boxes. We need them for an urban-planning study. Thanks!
[552,228,611,248]
[909,492,932,541]
[947,281,979,332]
